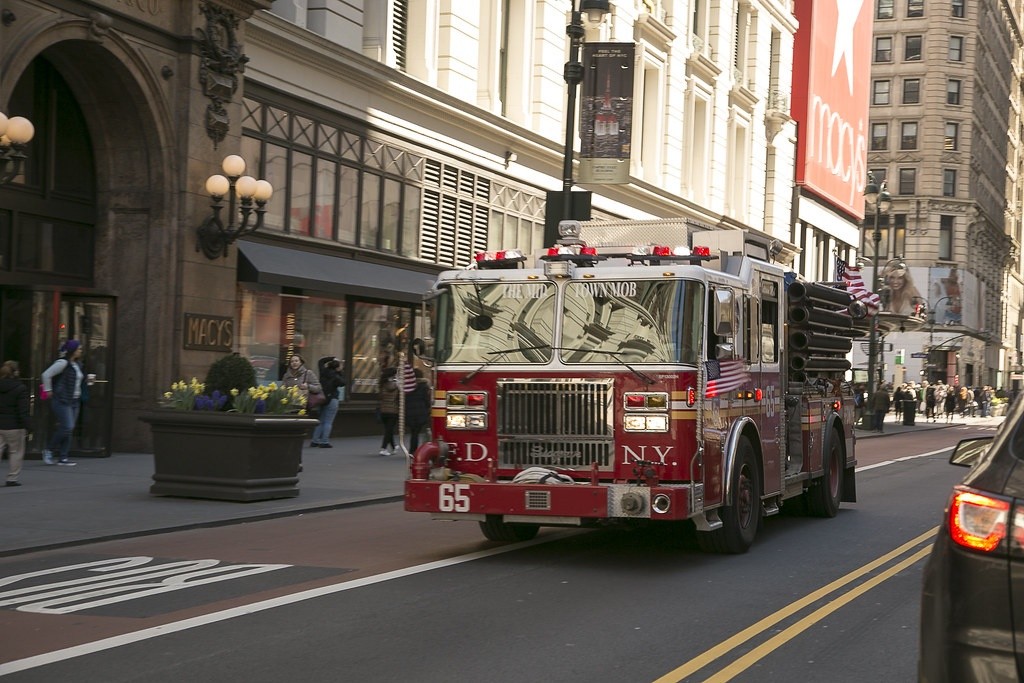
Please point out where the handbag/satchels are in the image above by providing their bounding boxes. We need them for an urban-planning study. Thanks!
[337,387,345,401]
[307,389,327,411]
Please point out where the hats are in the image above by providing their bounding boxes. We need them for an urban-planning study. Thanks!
[61,340,81,357]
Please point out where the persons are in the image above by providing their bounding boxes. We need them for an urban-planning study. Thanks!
[0,360,34,487]
[930,269,962,324]
[878,263,923,317]
[282,354,323,472]
[40,340,86,466]
[382,337,431,380]
[310,356,346,449]
[377,367,430,457]
[855,379,1020,434]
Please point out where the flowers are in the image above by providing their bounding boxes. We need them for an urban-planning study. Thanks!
[159,352,306,416]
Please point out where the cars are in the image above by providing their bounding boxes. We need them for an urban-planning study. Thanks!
[916,386,1023,681]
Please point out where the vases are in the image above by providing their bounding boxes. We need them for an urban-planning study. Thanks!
[137,410,320,503]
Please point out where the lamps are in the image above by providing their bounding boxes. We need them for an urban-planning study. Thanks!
[197,154,273,259]
[506,151,518,162]
[0,113,35,185]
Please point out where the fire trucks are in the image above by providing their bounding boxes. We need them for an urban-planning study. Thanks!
[403,216,869,554]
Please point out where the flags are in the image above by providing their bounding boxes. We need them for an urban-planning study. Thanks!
[835,257,880,317]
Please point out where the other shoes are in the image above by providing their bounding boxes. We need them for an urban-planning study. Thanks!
[311,442,320,447]
[392,445,400,455]
[5,481,20,486]
[379,449,391,456]
[320,443,333,448]
[409,452,414,459]
[42,447,54,465]
[57,459,76,466]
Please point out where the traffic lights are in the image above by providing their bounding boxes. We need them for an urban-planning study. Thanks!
[919,370,926,376]
[986,335,991,347]
[955,375,958,384]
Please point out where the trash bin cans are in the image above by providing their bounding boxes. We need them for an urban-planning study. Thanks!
[902,400,916,425]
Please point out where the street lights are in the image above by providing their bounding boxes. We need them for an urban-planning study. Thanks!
[562,1,611,217]
[955,352,960,385]
[863,170,894,429]
[913,295,956,383]
